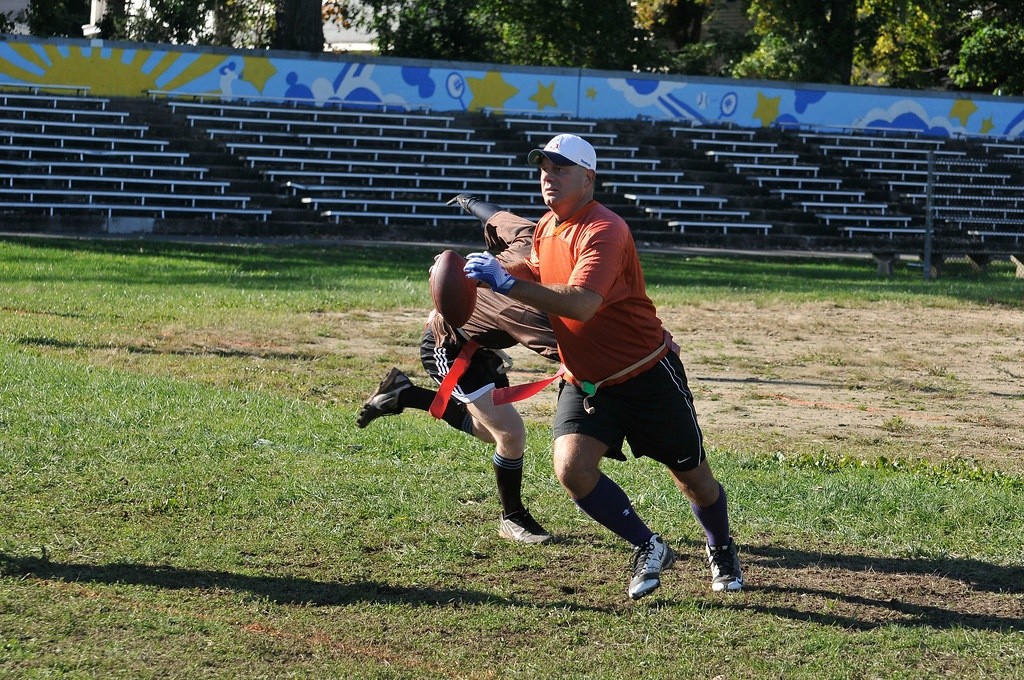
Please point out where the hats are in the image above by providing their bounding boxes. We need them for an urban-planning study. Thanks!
[528,134,596,171]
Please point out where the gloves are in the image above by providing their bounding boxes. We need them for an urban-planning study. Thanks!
[463,251,516,296]
[446,194,479,214]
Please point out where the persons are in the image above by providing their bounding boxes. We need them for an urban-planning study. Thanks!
[429,133,742,600]
[356,192,566,548]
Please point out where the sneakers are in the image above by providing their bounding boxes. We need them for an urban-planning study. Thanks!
[629,532,675,600]
[355,367,415,428]
[706,536,743,591]
[500,508,553,544]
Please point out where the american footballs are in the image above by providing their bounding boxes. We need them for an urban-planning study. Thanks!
[430,248,479,329]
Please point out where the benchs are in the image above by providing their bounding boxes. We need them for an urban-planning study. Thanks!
[664,122,1024,244]
[0,78,272,220]
[476,106,773,236]
[141,89,552,227]
